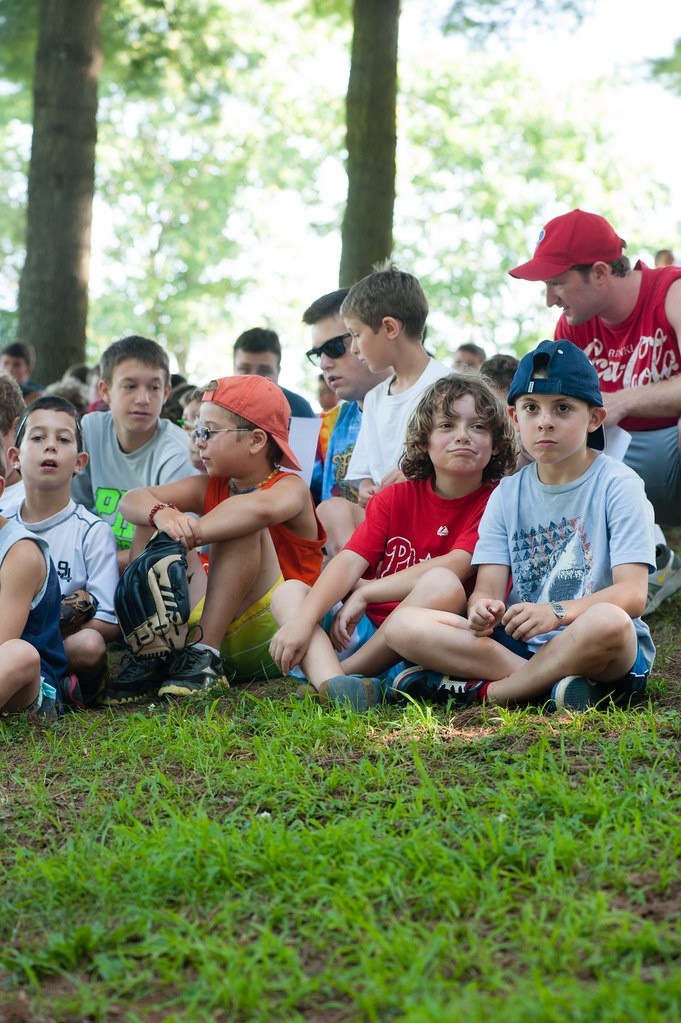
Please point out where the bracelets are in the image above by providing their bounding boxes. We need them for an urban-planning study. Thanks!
[148,502,176,530]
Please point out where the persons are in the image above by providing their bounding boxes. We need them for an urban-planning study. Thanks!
[0,208,681,722]
[267,374,522,714]
[98,374,327,706]
[508,208,681,520]
[0,394,121,688]
[313,257,460,558]
[301,289,395,504]
[383,340,657,714]
[69,334,202,575]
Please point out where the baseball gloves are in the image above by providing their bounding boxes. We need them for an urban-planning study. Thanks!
[56,591,97,633]
[113,529,192,660]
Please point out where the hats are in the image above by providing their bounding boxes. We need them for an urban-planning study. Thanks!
[201,374,302,471]
[508,208,627,281]
[506,339,606,451]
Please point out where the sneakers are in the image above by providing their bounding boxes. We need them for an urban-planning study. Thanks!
[318,674,383,711]
[551,675,603,712]
[391,665,489,710]
[157,624,231,702]
[60,672,84,713]
[640,543,681,616]
[295,683,318,700]
[93,643,173,707]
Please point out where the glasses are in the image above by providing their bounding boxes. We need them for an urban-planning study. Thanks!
[306,332,351,367]
[191,425,270,442]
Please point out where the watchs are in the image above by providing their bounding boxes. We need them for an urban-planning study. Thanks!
[547,600,566,621]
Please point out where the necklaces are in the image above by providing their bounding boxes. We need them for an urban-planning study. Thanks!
[227,466,281,495]
